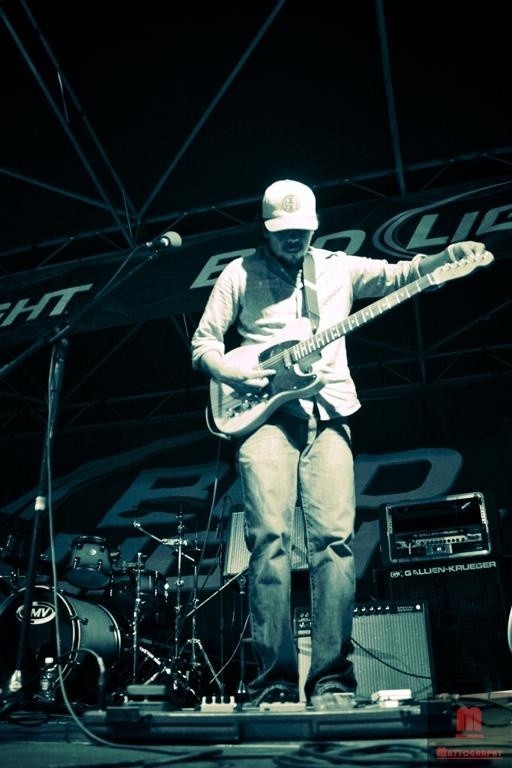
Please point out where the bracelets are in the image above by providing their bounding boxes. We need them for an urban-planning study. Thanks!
[447,243,457,262]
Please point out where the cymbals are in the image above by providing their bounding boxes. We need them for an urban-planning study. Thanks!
[3,554,64,575]
[0,512,42,540]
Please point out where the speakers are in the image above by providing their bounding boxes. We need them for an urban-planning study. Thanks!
[292,601,435,703]
[375,554,511,692]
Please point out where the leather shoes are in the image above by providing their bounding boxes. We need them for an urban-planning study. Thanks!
[264,689,294,703]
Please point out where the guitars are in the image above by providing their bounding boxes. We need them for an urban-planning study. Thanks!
[209,250,494,438]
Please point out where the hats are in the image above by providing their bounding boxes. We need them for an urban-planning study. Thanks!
[262,180,319,232]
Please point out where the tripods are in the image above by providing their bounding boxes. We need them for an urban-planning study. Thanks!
[0,257,86,726]
[131,537,222,699]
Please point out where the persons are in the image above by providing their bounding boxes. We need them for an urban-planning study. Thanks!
[191,179,487,705]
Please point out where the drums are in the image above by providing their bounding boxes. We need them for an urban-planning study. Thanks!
[0,585,121,689]
[66,536,113,590]
[109,568,170,604]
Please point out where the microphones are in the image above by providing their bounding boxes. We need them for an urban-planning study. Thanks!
[132,231,183,253]
